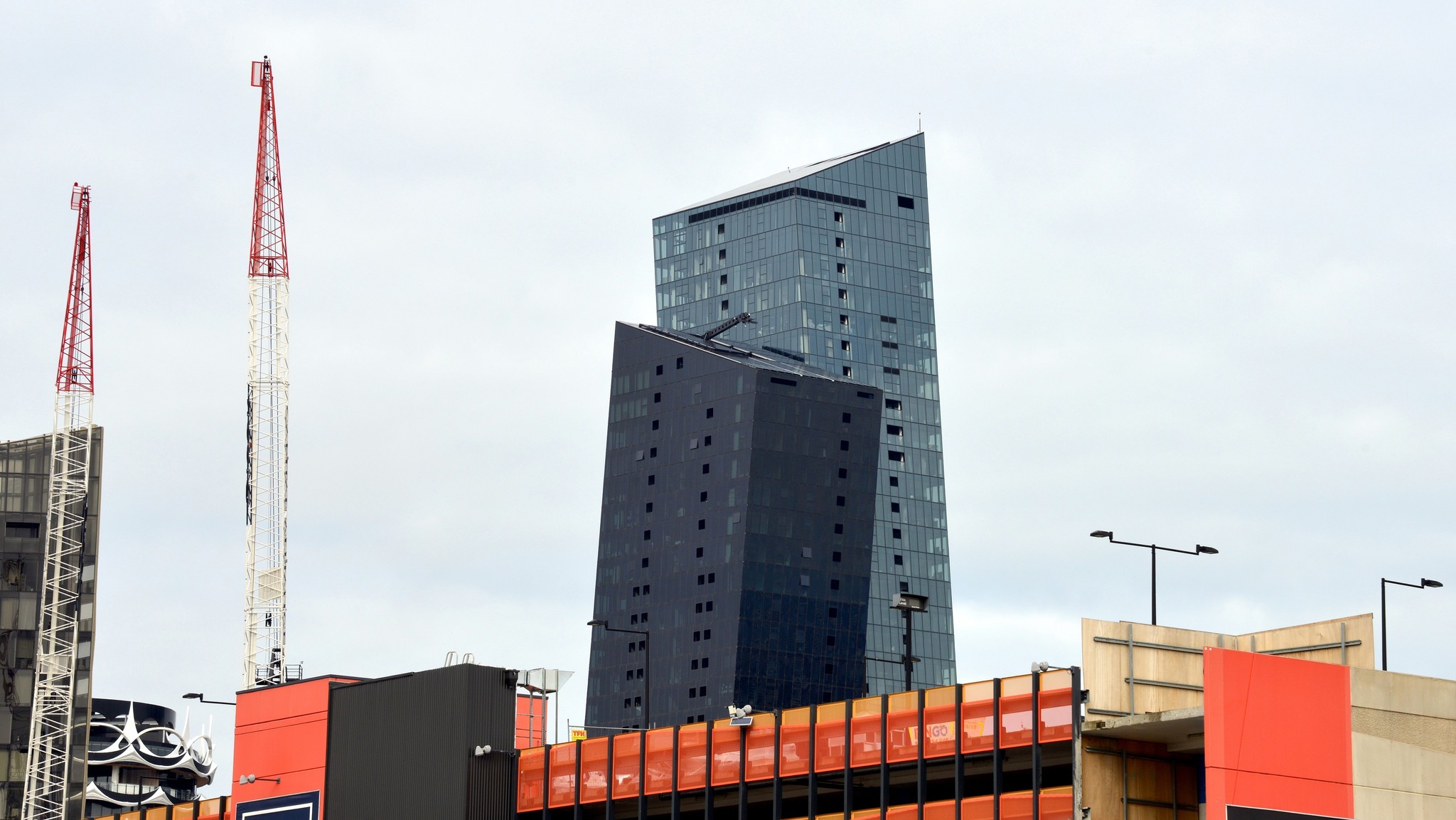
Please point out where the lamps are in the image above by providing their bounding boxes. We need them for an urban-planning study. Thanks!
[475,744,516,757]
[239,775,280,785]
[736,704,776,719]
[1031,661,1075,675]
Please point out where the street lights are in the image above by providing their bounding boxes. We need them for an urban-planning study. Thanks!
[587,618,651,732]
[1380,577,1444,671]
[890,593,930,690]
[1090,530,1220,626]
